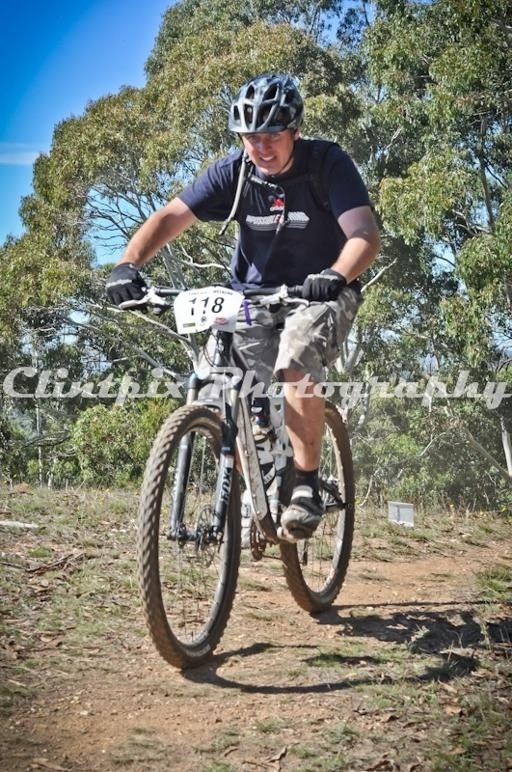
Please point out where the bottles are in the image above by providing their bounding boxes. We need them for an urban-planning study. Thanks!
[253,425,277,497]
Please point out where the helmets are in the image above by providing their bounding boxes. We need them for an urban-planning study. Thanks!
[227,74,304,134]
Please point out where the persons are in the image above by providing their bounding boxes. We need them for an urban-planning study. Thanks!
[98,69,384,542]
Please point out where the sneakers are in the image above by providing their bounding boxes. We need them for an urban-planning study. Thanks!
[279,481,326,541]
[240,487,255,551]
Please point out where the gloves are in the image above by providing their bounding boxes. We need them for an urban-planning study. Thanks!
[104,262,150,315]
[301,267,350,304]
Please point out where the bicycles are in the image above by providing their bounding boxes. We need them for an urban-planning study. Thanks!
[117,278,354,669]
